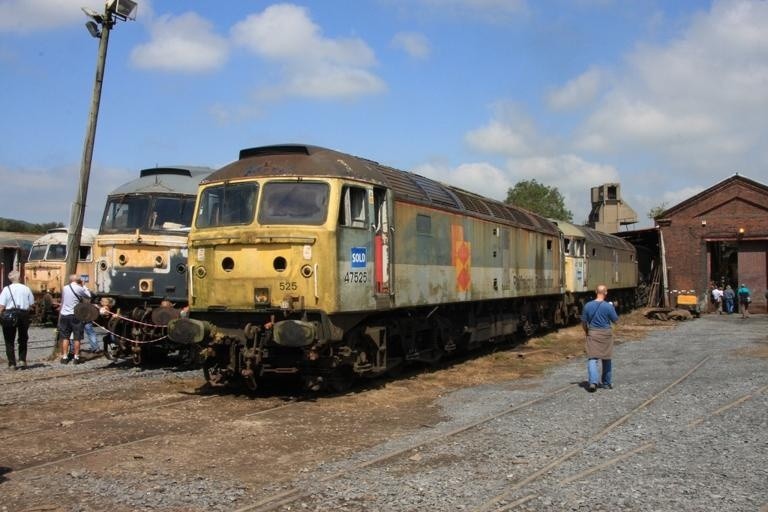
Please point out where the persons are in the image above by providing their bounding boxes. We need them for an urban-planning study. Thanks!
[1,271,34,370]
[70,323,99,355]
[60,274,92,363]
[717,287,724,302]
[738,284,752,319]
[723,284,735,313]
[584,286,617,392]
[712,286,722,316]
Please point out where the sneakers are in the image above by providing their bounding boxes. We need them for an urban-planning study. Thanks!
[60,357,80,365]
[589,384,614,392]
[8,360,27,370]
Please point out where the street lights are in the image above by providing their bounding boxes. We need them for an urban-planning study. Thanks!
[47,1,140,368]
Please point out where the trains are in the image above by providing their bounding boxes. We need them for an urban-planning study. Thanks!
[165,143,643,394]
[19,225,104,331]
[89,161,218,370]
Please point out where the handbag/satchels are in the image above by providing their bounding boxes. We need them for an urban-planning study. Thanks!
[0,309,18,327]
